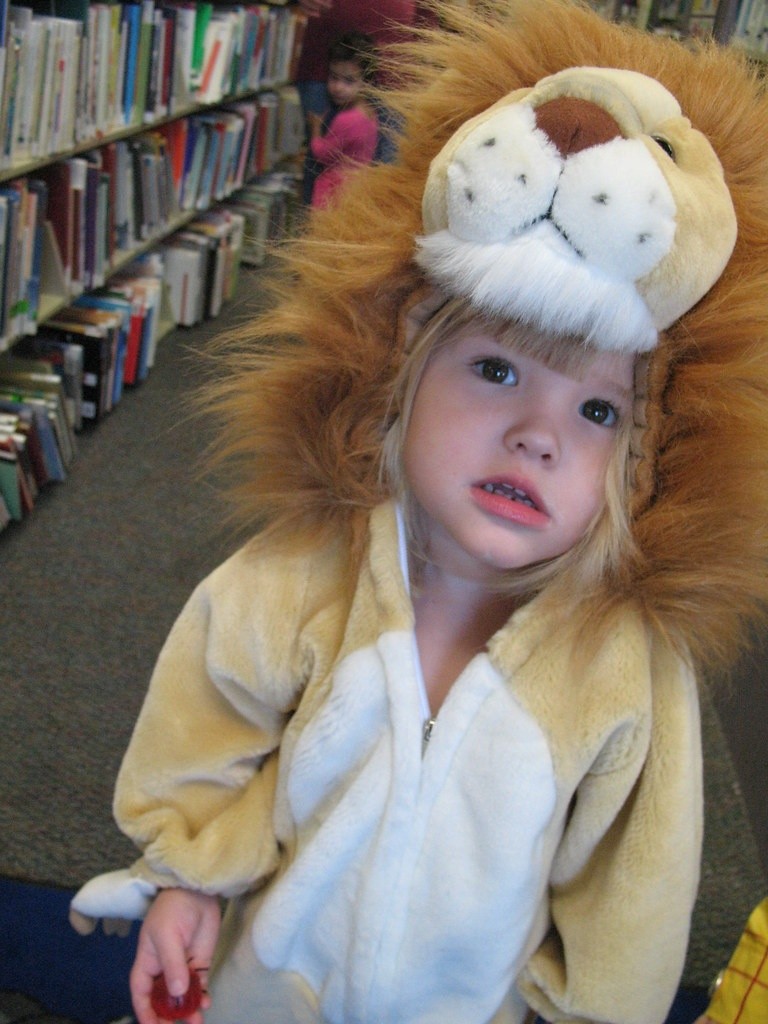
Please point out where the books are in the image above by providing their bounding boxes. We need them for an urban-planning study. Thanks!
[1,0,312,530]
[589,0,768,81]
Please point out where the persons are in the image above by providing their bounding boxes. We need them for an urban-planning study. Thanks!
[68,0,768,1024]
[310,35,379,210]
[284,0,442,201]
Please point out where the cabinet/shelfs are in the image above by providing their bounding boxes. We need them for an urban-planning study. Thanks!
[0,0,768,518]
